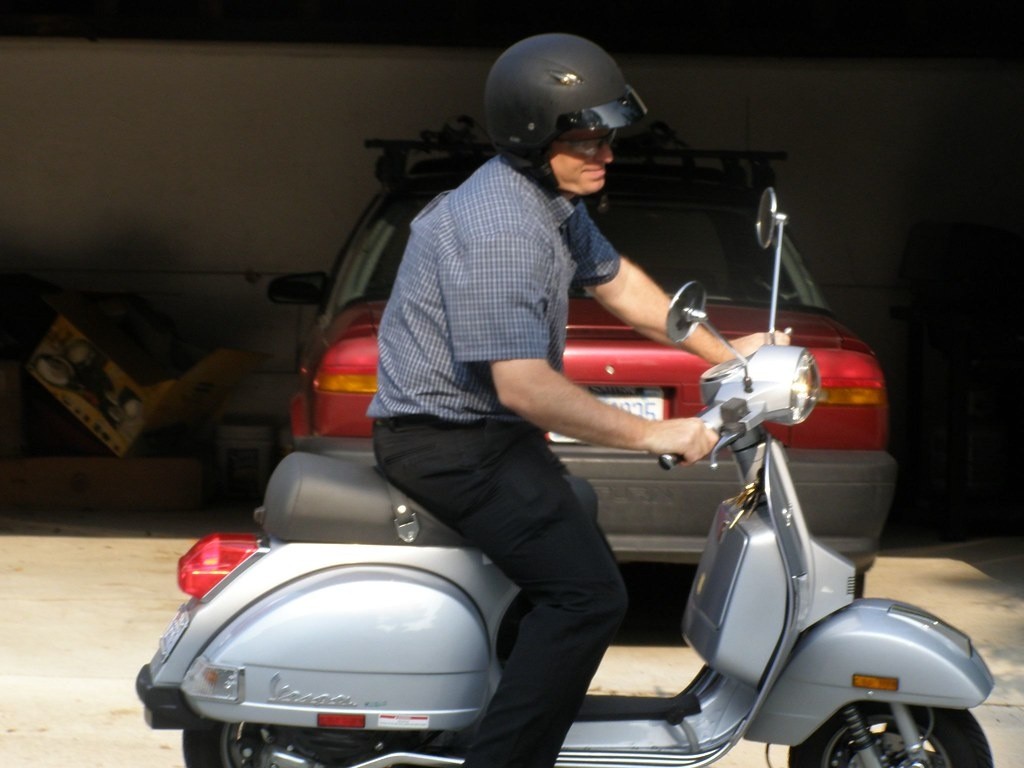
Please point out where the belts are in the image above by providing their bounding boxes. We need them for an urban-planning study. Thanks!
[372,410,437,430]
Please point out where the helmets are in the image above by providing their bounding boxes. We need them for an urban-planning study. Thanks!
[483,34,647,171]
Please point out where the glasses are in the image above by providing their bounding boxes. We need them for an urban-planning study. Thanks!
[552,125,616,160]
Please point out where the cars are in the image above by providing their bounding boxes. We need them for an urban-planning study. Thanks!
[264,142,897,631]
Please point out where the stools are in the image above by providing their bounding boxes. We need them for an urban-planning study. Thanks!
[212,424,278,499]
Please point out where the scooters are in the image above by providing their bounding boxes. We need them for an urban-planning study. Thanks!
[136,185,996,768]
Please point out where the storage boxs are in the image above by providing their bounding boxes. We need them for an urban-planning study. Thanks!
[898,221,1024,292]
[24,290,275,457]
[0,360,27,456]
[0,456,221,514]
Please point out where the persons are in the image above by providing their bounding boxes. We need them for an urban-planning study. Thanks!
[367,32,790,767]
